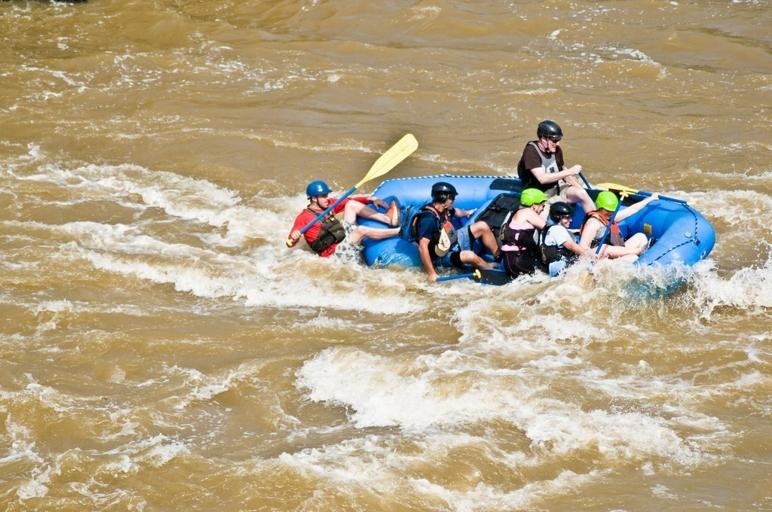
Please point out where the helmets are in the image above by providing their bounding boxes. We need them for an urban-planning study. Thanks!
[520,188,547,207]
[306,180,332,196]
[595,191,618,212]
[431,182,458,197]
[537,120,563,139]
[550,202,572,223]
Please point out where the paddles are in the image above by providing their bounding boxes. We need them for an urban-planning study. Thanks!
[286,133,418,247]
[597,182,687,204]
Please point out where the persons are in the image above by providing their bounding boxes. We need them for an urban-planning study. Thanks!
[501,187,548,281]
[540,202,599,279]
[286,179,402,259]
[517,120,598,214]
[412,182,503,283]
[578,190,661,273]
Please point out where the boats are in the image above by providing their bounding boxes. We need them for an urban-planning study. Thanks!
[337,172,717,302]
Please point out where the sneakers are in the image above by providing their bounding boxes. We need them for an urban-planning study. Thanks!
[386,200,400,227]
[646,237,655,249]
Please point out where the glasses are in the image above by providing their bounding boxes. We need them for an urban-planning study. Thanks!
[550,138,560,143]
[541,203,545,208]
[448,195,455,199]
[563,215,572,219]
